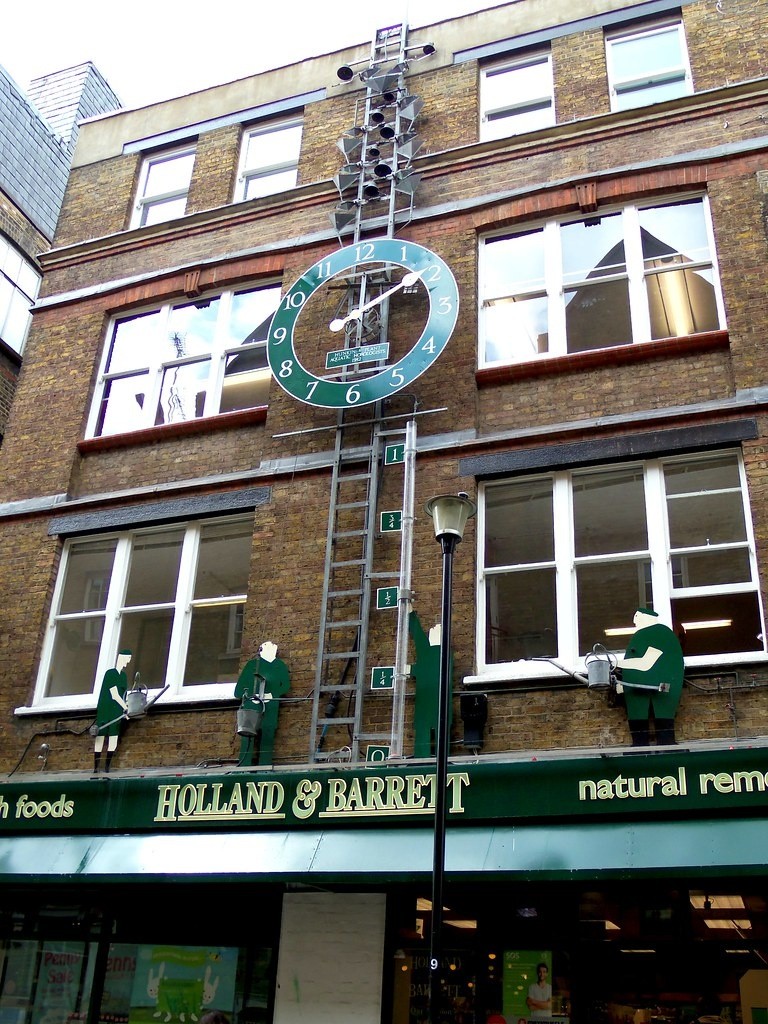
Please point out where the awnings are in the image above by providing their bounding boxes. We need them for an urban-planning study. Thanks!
[0,817,767,884]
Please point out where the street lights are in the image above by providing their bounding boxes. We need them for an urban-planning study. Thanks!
[428,492,478,1024]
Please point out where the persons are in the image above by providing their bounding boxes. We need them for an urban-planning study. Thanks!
[527,963,552,1017]
